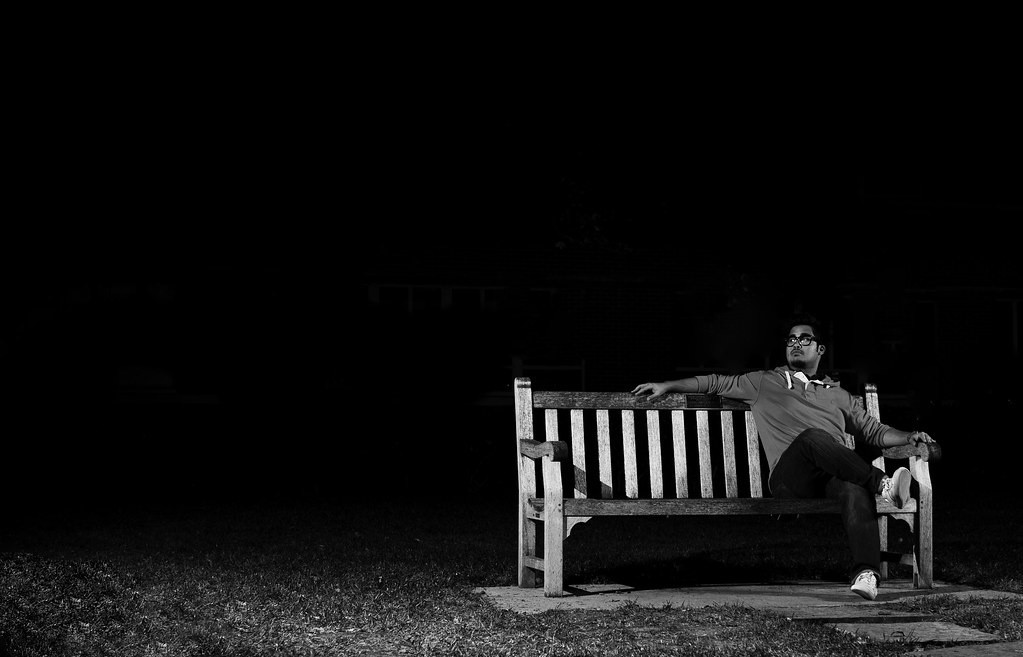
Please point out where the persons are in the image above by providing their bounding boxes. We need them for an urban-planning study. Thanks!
[630,313,936,600]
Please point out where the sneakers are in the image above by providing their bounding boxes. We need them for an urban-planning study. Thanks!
[881,467,911,509]
[850,572,878,600]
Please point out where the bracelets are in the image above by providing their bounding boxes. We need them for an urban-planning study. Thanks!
[907,431,918,442]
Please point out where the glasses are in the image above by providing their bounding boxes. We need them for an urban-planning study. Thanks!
[785,334,819,347]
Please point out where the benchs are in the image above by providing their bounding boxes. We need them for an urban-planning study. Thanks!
[514,375,933,596]
